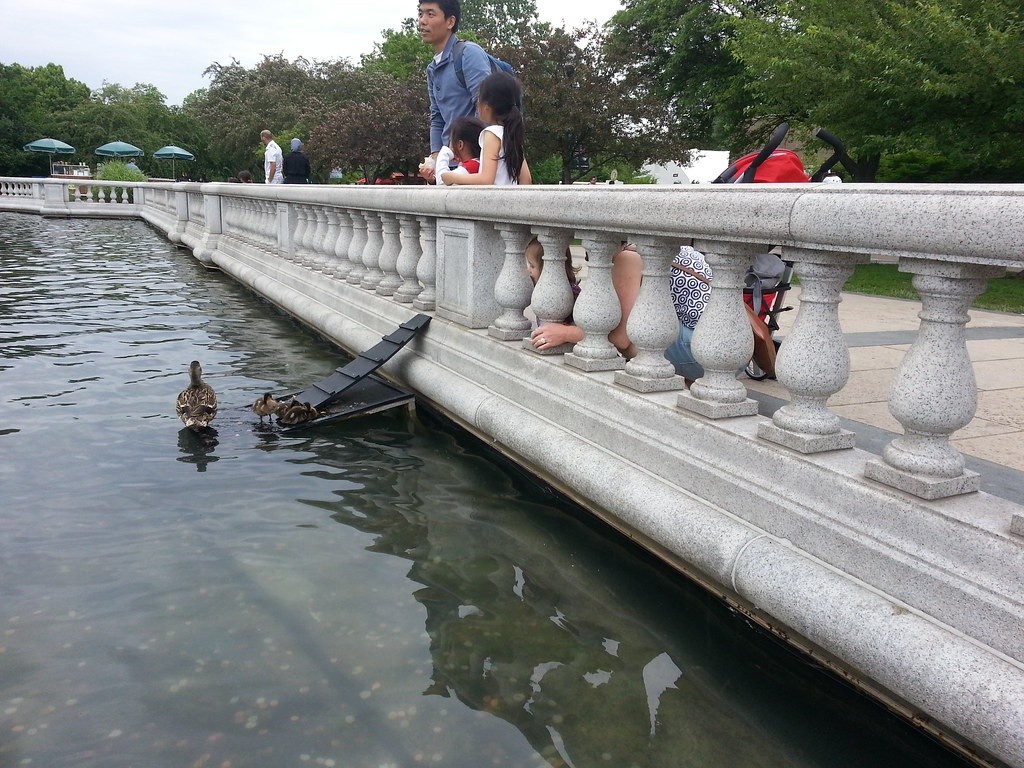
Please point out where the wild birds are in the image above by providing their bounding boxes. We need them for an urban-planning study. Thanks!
[253,393,317,424]
[176,361,217,432]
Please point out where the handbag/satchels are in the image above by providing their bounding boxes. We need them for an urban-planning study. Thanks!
[744,302,775,378]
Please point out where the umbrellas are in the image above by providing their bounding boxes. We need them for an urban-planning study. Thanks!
[153,145,197,180]
[95,140,144,172]
[23,137,76,177]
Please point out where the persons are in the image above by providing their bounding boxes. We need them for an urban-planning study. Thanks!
[260,130,283,184]
[284,139,310,183]
[525,238,712,382]
[125,158,140,172]
[239,171,252,183]
[418,0,532,185]
[587,177,597,185]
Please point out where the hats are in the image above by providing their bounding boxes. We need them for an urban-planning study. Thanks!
[291,138,301,153]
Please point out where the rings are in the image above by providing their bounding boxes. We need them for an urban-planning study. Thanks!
[541,338,546,344]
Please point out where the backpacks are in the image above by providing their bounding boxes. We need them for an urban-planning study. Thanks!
[453,38,514,93]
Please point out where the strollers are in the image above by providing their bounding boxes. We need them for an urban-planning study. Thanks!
[690,122,846,381]
